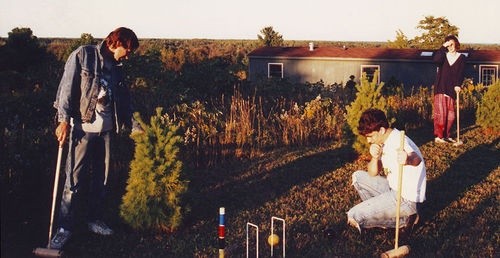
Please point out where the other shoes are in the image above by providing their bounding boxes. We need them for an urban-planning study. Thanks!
[446,137,456,143]
[434,136,447,144]
[398,212,419,238]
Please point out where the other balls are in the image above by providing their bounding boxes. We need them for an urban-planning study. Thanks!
[269,233,278,245]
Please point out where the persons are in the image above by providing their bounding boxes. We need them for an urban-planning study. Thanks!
[433,35,474,145]
[47,27,139,249]
[346,108,427,244]
[344,75,359,96]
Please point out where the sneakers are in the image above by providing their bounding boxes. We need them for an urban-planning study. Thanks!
[51,228,73,249]
[88,220,114,235]
